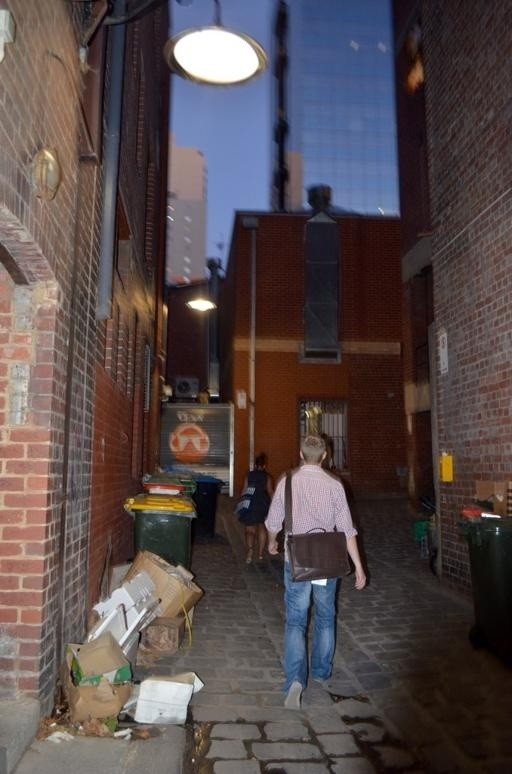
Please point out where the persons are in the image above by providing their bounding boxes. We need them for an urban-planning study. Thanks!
[264,435,367,708]
[242,454,274,564]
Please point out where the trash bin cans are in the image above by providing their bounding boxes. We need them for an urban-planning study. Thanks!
[191,475,226,545]
[456,518,512,671]
[124,493,194,571]
[137,472,198,497]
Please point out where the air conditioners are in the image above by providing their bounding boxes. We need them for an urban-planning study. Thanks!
[173,376,199,398]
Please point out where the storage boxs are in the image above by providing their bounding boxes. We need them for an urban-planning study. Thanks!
[475,478,507,519]
[64,547,203,728]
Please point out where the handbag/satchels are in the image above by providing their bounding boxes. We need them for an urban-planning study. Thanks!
[234,487,256,514]
[286,531,351,582]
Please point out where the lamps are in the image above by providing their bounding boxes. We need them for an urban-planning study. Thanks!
[74,0,265,86]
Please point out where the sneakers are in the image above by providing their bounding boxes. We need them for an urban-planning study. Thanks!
[259,550,265,560]
[246,547,253,564]
[284,681,303,710]
